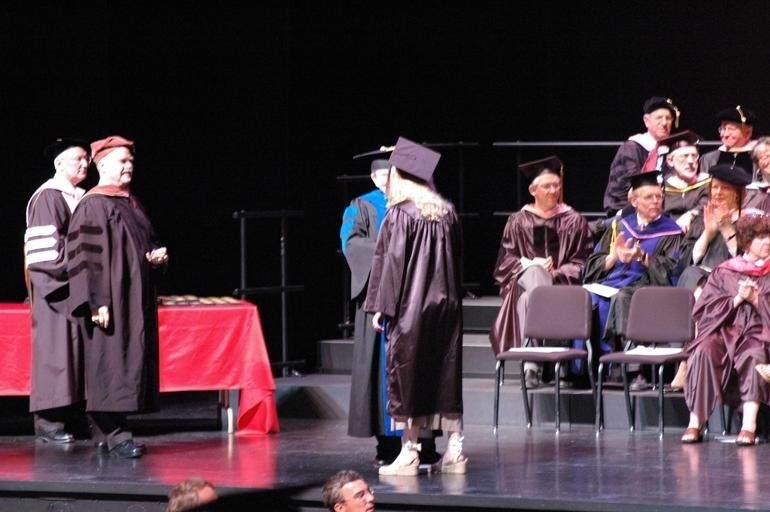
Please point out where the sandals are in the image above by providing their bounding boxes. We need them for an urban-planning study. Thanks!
[735,430,755,446]
[681,421,704,443]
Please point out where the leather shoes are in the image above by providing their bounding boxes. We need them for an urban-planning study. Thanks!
[41,429,74,443]
[94,441,147,457]
[523,370,683,394]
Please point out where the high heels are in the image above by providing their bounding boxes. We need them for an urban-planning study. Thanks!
[374,433,469,479]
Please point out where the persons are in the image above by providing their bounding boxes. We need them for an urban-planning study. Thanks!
[377,467,469,497]
[364,134,468,475]
[679,210,769,446]
[20,135,93,442]
[669,443,763,507]
[337,150,443,468]
[570,98,770,393]
[165,479,218,511]
[489,153,593,389]
[64,135,169,459]
[321,469,376,512]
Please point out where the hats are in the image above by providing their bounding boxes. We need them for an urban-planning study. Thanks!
[624,97,754,189]
[90,135,134,165]
[354,136,441,183]
[518,156,565,204]
[40,136,91,177]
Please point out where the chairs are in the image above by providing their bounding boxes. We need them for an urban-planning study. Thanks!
[492,284,728,438]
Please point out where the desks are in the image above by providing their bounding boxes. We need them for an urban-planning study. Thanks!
[0,303,280,436]
